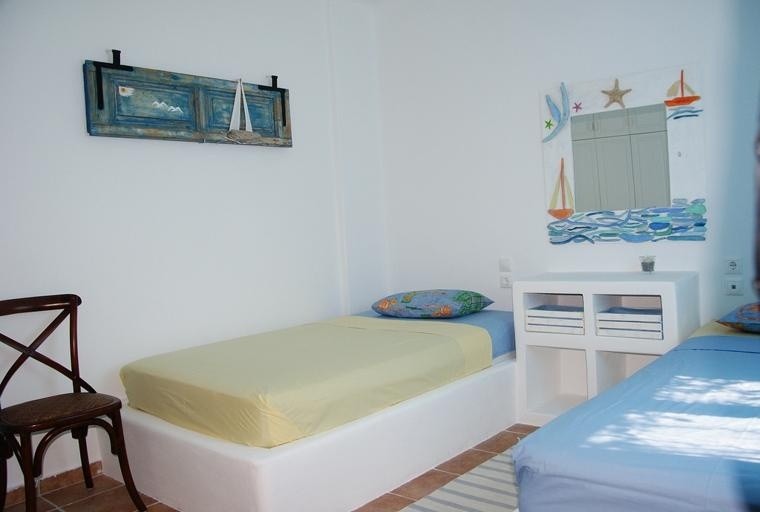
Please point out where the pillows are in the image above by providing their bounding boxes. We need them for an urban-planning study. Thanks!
[372,287,496,316]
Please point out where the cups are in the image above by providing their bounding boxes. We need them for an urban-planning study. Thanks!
[639,254,657,273]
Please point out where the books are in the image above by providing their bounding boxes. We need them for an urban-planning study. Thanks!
[526,304,584,335]
[596,306,663,340]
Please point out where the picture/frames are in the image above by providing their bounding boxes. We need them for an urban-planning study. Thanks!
[81,58,293,149]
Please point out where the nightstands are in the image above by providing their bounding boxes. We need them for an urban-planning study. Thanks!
[515,272,700,427]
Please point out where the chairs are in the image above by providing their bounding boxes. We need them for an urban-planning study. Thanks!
[0,292,149,512]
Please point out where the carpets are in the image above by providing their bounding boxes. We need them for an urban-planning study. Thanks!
[400,438,518,512]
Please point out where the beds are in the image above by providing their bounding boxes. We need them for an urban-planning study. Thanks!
[96,306,517,512]
[486,317,758,511]
